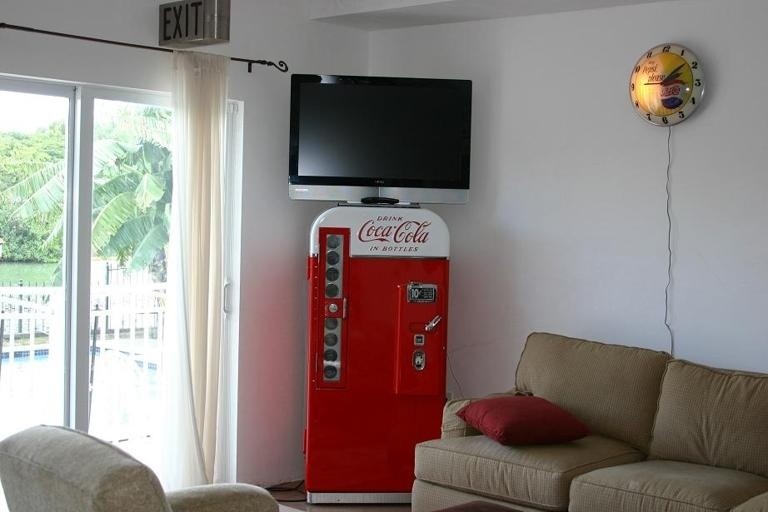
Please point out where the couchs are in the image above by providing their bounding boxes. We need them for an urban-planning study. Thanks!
[409,331,766,512]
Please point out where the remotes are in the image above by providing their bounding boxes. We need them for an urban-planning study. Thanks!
[360,197,399,205]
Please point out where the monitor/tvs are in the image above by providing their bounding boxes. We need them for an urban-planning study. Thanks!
[288,73,472,208]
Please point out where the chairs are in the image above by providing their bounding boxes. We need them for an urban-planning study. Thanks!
[0,424,283,512]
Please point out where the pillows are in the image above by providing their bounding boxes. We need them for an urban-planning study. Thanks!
[455,396,593,448]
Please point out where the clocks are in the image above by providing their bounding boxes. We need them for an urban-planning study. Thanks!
[628,43,706,127]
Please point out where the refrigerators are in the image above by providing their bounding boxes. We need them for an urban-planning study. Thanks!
[301,204,450,506]
